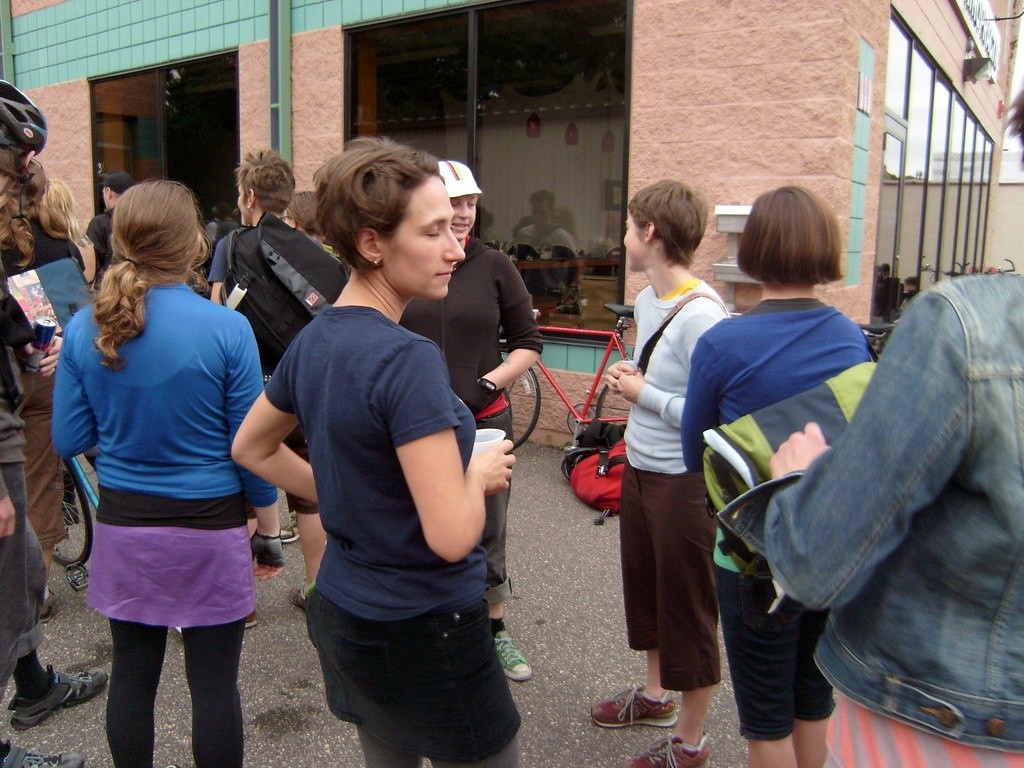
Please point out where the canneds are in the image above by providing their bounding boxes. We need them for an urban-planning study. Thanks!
[24,316,58,373]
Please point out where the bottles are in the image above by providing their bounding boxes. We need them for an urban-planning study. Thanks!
[539,242,553,261]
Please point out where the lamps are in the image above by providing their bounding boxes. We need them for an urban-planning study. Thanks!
[527,92,614,155]
[962,58,996,84]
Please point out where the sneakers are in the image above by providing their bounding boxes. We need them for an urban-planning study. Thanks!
[0,745,83,768]
[9,664,108,729]
[624,730,710,768]
[592,685,678,727]
[494,631,531,680]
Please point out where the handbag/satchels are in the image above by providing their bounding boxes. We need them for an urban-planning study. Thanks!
[568,421,633,524]
[702,361,879,577]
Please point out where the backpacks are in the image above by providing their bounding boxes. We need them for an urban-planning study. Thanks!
[219,212,351,374]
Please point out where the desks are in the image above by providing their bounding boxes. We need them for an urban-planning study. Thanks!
[513,255,621,284]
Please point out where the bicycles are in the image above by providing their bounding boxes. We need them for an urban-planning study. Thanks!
[494,302,635,453]
[49,419,102,567]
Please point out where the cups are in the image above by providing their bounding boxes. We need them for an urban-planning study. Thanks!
[472,428,506,456]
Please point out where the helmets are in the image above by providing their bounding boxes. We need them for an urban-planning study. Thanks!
[0,79,48,156]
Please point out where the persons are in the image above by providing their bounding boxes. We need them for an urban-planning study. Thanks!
[873,259,922,322]
[717,73,1024,768]
[515,190,580,298]
[208,147,351,628]
[591,177,870,768]
[50,177,287,768]
[0,176,87,277]
[398,159,544,680]
[85,172,135,291]
[0,80,107,768]
[508,244,568,305]
[231,135,521,768]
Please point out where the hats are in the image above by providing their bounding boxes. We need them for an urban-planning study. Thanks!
[437,160,482,198]
[98,172,136,194]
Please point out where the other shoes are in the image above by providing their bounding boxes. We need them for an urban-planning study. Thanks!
[289,583,309,609]
[279,524,300,543]
[39,589,56,622]
